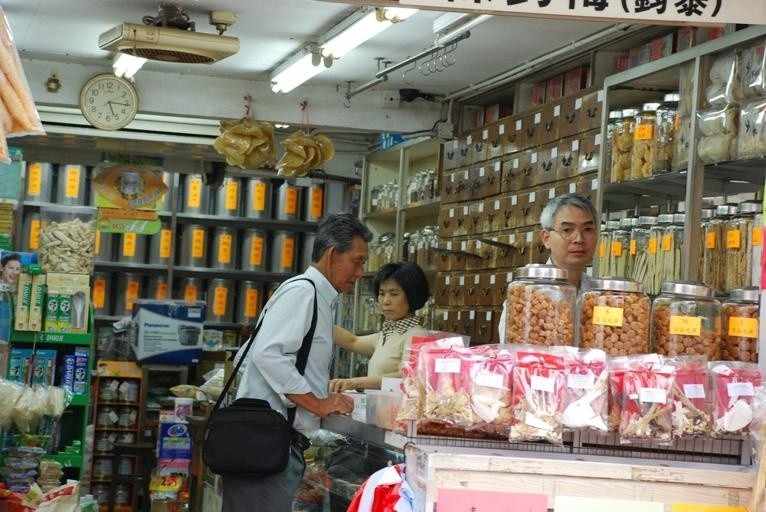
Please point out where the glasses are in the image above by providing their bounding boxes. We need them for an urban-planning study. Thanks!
[547,226,598,245]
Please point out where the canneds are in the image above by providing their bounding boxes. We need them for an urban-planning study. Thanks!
[89,378,140,511]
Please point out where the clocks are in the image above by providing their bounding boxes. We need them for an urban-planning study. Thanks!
[77,71,140,133]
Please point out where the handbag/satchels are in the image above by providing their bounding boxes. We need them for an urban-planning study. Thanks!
[202,398,290,477]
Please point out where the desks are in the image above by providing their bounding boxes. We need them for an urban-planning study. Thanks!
[297,389,766,512]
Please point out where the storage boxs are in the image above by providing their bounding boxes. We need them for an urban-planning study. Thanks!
[129,301,208,368]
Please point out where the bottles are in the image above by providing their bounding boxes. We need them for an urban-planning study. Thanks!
[505,202,763,364]
[23,161,324,351]
[607,92,679,183]
[368,169,438,272]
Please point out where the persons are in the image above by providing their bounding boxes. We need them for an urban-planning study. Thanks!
[496,194,598,345]
[223,210,373,512]
[329,260,430,393]
[0,253,23,289]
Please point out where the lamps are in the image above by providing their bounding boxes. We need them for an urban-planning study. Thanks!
[432,12,495,47]
[111,48,149,82]
[268,3,422,102]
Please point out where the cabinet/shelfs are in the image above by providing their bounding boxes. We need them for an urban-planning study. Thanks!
[346,25,766,381]
[15,156,331,377]
[0,301,193,512]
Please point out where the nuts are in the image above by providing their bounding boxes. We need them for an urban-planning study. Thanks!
[505,284,760,363]
